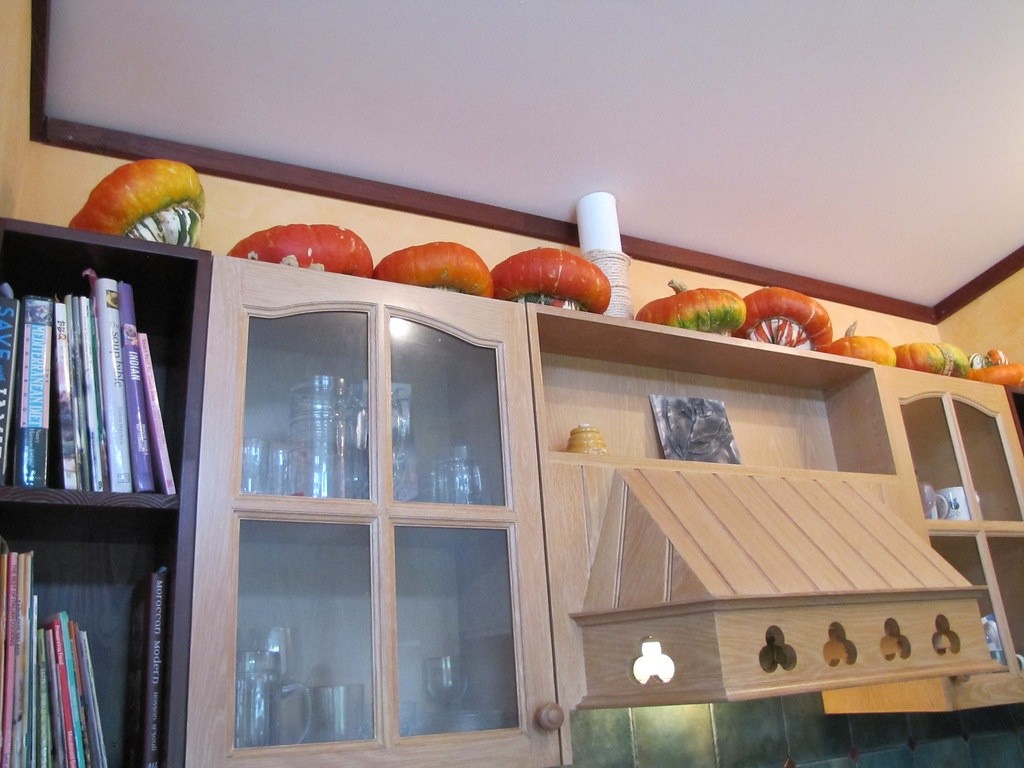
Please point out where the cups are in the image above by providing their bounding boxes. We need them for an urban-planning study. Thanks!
[302,684,365,740]
[936,486,980,521]
[424,654,469,712]
[235,651,311,747]
[247,627,295,697]
[435,443,483,505]
[990,649,1024,671]
[241,438,296,496]
[919,481,950,520]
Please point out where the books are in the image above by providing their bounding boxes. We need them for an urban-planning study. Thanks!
[122,565,167,768]
[0,267,176,494]
[0,552,108,768]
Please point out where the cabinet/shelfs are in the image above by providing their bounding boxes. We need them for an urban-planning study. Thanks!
[0,213,1024,768]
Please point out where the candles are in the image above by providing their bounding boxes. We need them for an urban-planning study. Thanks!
[576,192,622,254]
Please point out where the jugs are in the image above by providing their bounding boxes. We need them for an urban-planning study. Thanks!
[290,374,417,501]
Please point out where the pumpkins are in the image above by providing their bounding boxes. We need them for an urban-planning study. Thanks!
[69,159,1024,385]
